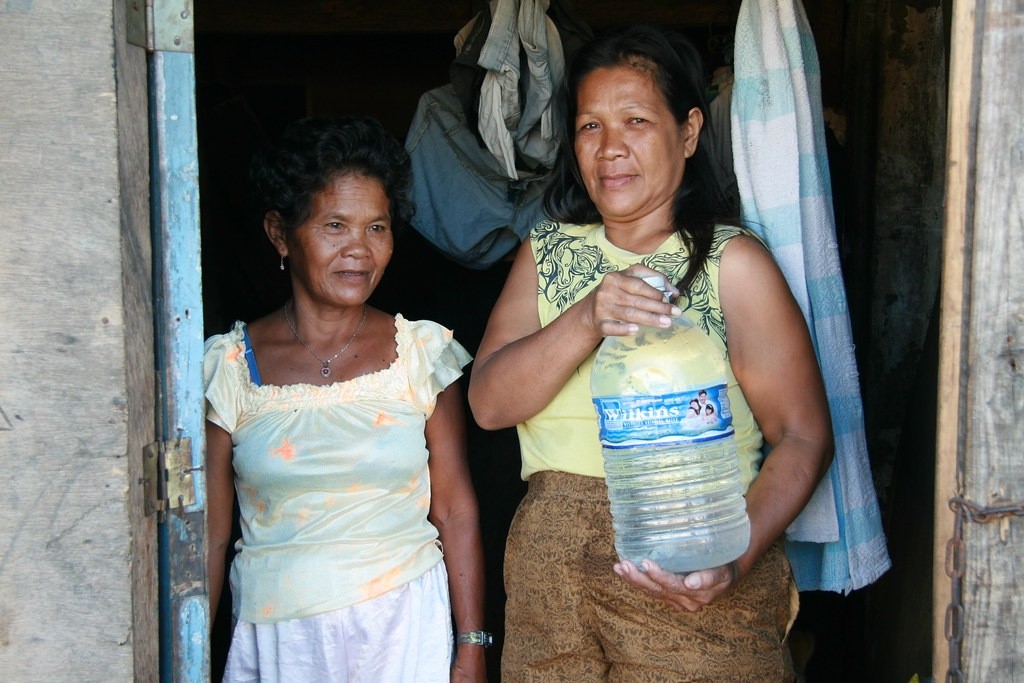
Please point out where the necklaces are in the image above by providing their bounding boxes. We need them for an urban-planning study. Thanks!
[282,302,367,379]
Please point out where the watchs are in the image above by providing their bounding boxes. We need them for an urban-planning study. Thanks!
[454,632,493,651]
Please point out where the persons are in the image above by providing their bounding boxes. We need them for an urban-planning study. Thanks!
[685,390,718,427]
[201,118,488,683]
[466,31,836,683]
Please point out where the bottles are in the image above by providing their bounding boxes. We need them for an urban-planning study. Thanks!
[587,276,753,574]
